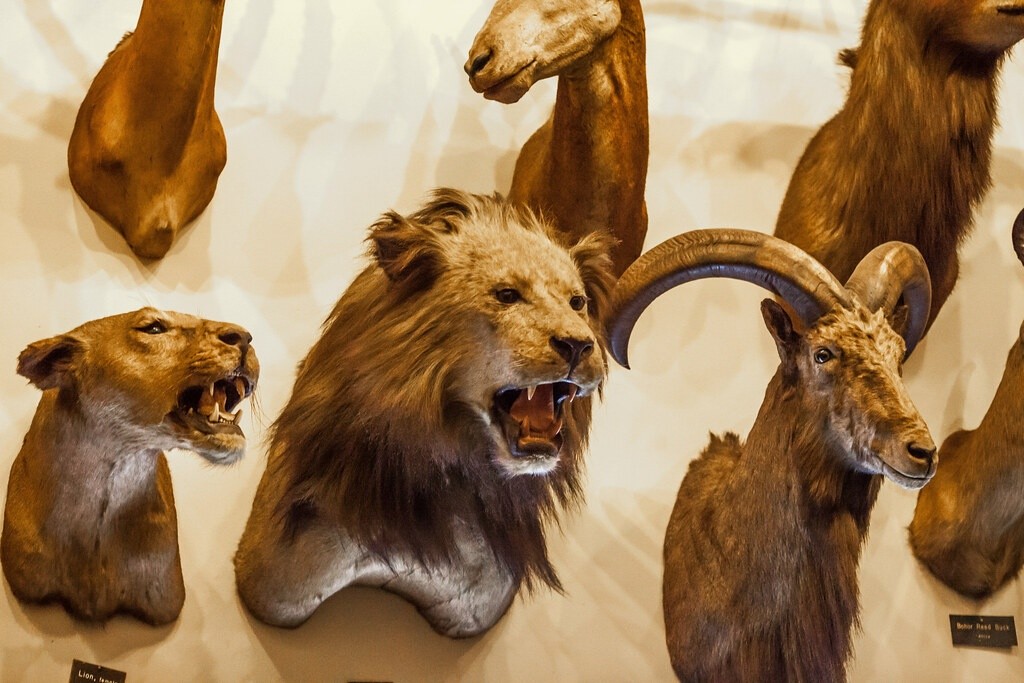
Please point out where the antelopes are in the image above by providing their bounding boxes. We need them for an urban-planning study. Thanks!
[602,228,940,682]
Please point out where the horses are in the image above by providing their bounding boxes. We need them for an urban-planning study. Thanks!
[463,0,649,277]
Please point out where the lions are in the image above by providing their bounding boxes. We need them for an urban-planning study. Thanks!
[232,187,622,637]
